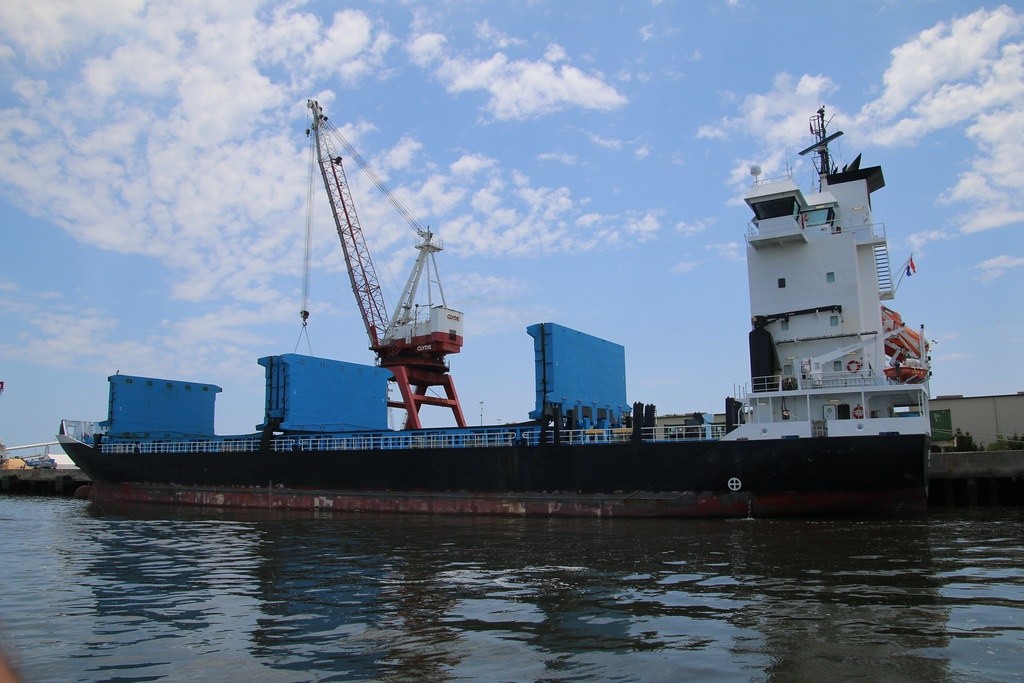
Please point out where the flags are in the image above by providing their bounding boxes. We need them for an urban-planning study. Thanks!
[906,257,916,277]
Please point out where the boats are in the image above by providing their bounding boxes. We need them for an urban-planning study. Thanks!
[54,323,930,521]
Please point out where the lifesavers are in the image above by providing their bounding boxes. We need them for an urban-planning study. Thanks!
[846,359,862,372]
[852,406,865,421]
